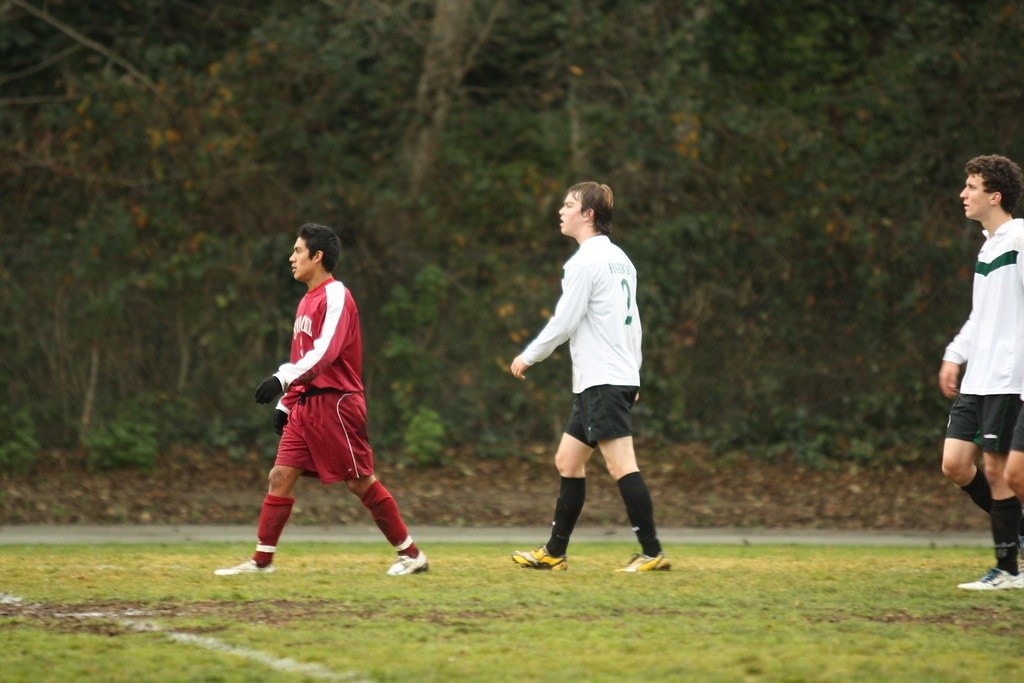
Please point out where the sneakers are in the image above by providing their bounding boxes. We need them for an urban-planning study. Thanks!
[617,551,671,574]
[386,550,429,576]
[213,559,275,575]
[956,568,1024,592]
[512,546,567,571]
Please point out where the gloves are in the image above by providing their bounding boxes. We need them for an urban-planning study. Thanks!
[272,409,288,436]
[253,376,283,405]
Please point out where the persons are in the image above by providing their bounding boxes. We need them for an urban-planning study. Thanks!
[510,182,671,572]
[215,224,428,575]
[937,154,1024,590]
